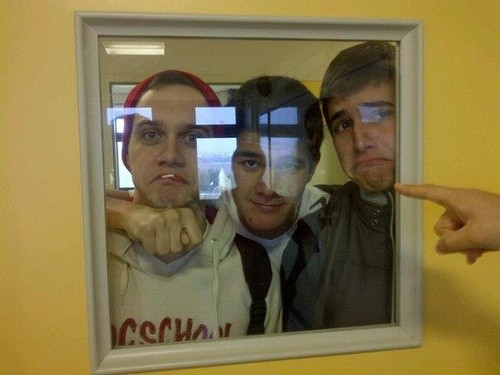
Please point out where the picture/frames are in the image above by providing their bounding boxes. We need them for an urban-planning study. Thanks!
[75,9,425,374]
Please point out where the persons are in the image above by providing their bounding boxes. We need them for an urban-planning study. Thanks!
[396,182,500,264]
[102,69,284,355]
[222,76,334,272]
[279,40,396,332]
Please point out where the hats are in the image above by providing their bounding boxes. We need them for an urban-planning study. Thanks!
[119,70,225,180]
